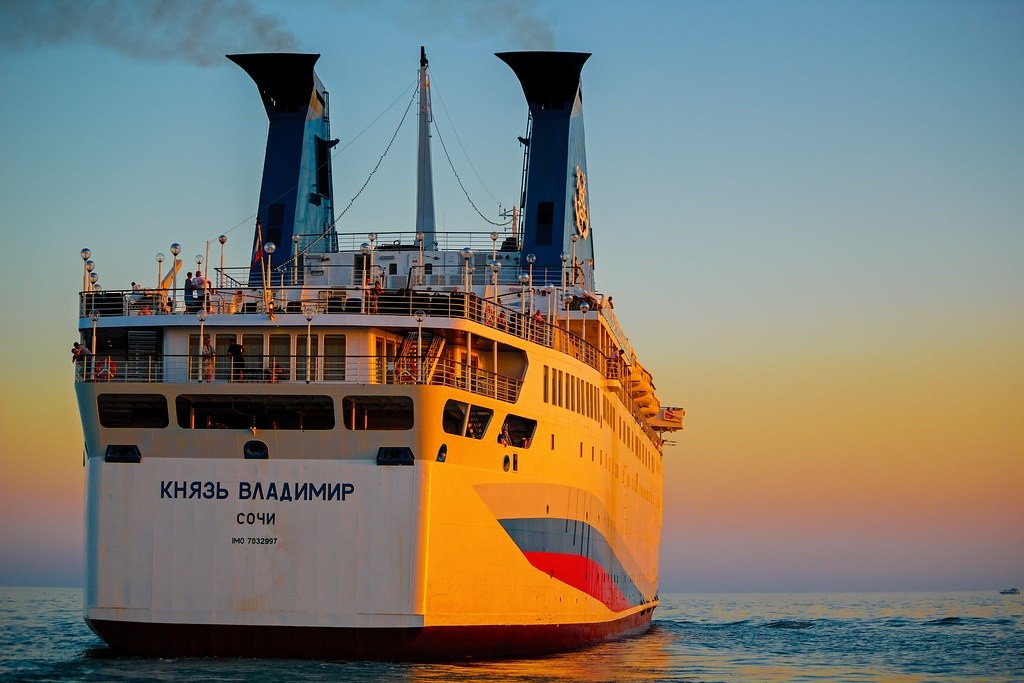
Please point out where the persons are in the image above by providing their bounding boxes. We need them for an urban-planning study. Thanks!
[155,303,168,315]
[71,342,91,381]
[533,310,545,326]
[202,338,217,383]
[266,360,282,380]
[185,271,211,299]
[138,304,152,315]
[226,338,245,383]
[608,296,614,309]
[131,282,140,290]
[366,278,384,295]
[497,298,510,323]
[497,429,508,445]
[167,297,173,307]
[611,349,624,361]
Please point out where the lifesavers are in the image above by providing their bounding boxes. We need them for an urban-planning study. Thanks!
[483,306,496,326]
[395,361,417,381]
[97,359,117,379]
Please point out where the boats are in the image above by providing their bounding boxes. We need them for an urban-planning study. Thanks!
[78,50,686,665]
